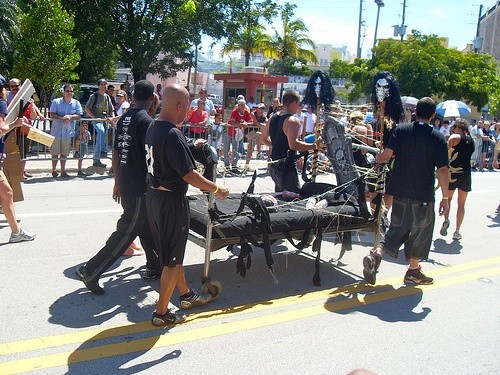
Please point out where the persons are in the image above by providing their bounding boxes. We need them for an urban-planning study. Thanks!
[262,89,323,203]
[49,83,83,178]
[154,84,163,114]
[145,83,229,326]
[77,80,208,295]
[75,120,91,176]
[430,117,450,137]
[107,84,132,122]
[184,89,282,174]
[440,119,475,240]
[85,79,116,168]
[295,104,316,137]
[363,97,450,285]
[0,74,45,242]
[469,119,500,172]
[334,107,394,227]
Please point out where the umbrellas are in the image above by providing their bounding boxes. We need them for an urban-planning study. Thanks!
[401,96,419,109]
[436,100,471,117]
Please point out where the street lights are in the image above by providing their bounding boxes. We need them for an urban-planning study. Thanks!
[371,0,385,60]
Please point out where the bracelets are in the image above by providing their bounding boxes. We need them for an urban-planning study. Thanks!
[214,186,218,194]
[443,197,448,199]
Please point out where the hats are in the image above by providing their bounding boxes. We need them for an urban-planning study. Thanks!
[98,79,107,85]
[78,121,87,126]
[116,90,127,97]
[252,103,265,109]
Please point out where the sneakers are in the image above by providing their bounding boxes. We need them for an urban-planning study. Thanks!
[403,265,434,285]
[179,290,213,309]
[9,228,36,243]
[363,250,382,285]
[144,267,163,276]
[448,231,462,239]
[75,264,105,295]
[440,219,450,236]
[151,308,186,325]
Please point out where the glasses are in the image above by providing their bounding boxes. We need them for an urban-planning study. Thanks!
[11,85,19,88]
[65,90,73,92]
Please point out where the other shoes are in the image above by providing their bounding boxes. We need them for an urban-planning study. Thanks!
[93,161,107,168]
[52,172,59,178]
[61,172,69,177]
[78,171,86,176]
